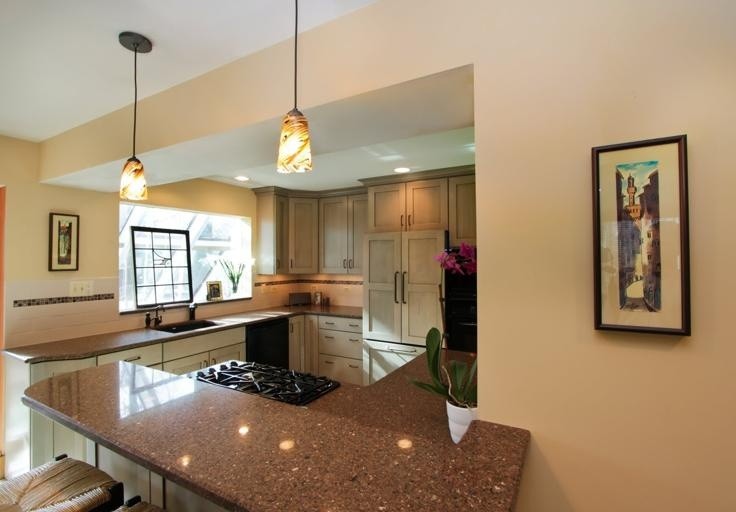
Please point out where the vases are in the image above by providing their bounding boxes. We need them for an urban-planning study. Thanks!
[445,397,477,447]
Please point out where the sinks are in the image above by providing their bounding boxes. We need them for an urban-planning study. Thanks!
[157,319,219,334]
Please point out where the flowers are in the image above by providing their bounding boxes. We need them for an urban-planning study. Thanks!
[401,245,479,408]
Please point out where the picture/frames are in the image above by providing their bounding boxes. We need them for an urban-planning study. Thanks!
[47,213,79,272]
[207,283,223,299]
[590,135,690,339]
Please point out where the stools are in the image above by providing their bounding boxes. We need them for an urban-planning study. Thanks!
[1,455,123,512]
[111,495,160,512]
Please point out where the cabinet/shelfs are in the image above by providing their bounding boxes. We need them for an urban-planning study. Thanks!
[361,340,427,387]
[369,174,450,231]
[317,186,368,276]
[1,355,95,484]
[447,180,476,243]
[290,315,308,375]
[250,188,318,277]
[163,327,246,374]
[304,314,363,386]
[362,231,449,347]
[96,342,163,370]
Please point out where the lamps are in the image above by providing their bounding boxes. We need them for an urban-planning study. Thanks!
[273,1,313,176]
[117,31,153,201]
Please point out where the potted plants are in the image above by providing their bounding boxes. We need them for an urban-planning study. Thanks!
[219,257,247,298]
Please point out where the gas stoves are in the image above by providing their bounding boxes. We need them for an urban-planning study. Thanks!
[181,358,340,406]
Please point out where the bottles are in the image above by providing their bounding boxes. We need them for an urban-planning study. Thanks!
[322,297,329,306]
[314,291,321,306]
[188,302,197,320]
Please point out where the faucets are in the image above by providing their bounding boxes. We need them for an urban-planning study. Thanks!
[144,305,165,328]
